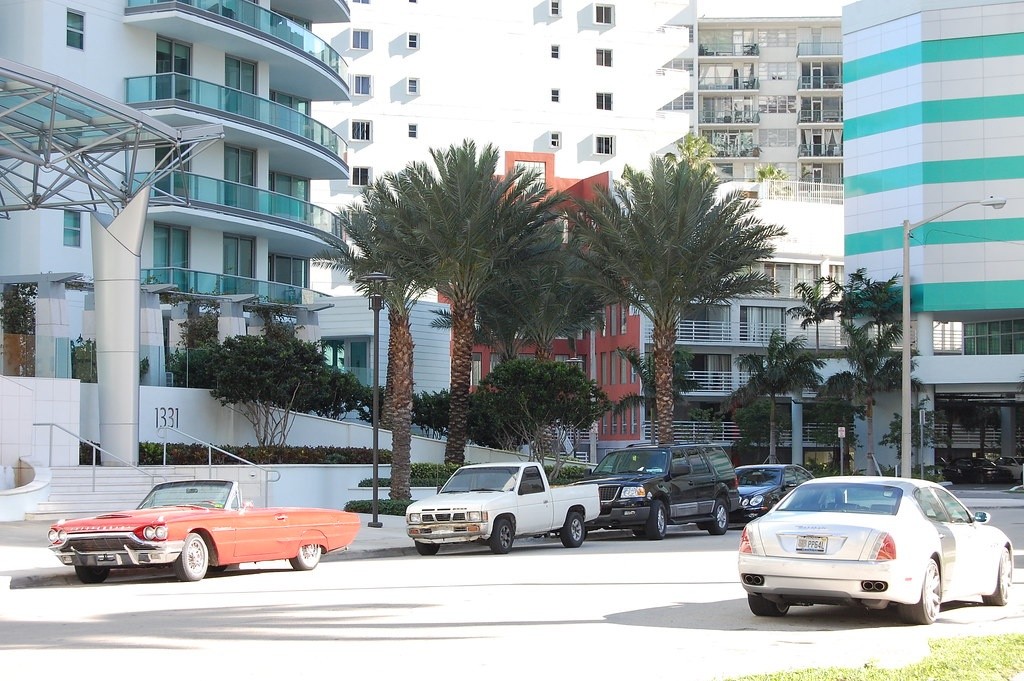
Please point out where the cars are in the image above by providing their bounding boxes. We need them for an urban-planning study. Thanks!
[942,456,1014,484]
[994,456,1024,482]
[696,463,816,531]
[738,473,1015,626]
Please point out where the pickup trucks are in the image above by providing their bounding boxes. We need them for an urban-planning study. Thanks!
[404,461,601,556]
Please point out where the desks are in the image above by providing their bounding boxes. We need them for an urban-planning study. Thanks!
[824,117,839,121]
[716,52,733,56]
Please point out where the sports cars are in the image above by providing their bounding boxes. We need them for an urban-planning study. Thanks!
[46,478,361,585]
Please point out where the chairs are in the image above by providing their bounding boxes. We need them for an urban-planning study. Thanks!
[705,49,714,55]
[724,116,732,122]
[832,147,841,156]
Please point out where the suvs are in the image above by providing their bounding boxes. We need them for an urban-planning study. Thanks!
[567,441,740,540]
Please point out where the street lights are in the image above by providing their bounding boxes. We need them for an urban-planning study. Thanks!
[901,194,1007,478]
[564,356,586,460]
[357,271,397,529]
